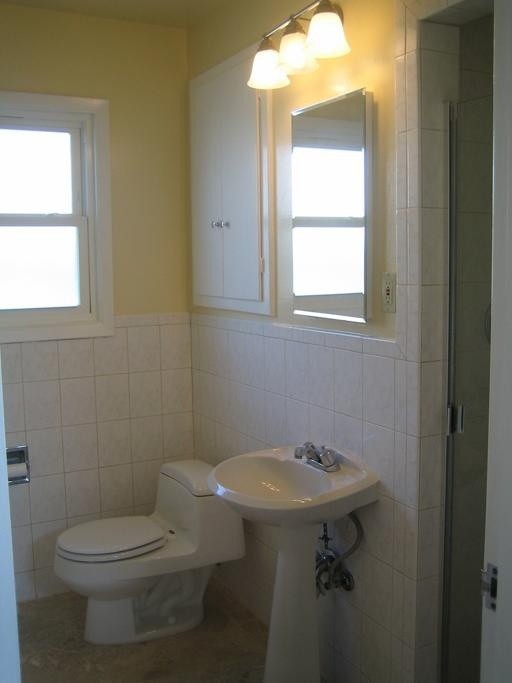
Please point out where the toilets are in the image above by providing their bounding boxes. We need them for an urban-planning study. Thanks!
[53,459,243,645]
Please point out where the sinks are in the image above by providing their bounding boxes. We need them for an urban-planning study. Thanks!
[207,444,380,527]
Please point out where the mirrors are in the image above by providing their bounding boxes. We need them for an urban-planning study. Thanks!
[287,90,371,329]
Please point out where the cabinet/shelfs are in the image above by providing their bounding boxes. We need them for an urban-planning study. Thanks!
[187,47,264,304]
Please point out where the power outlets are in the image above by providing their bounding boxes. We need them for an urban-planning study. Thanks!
[381,269,397,313]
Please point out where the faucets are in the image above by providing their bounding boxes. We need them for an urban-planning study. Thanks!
[294,445,319,464]
